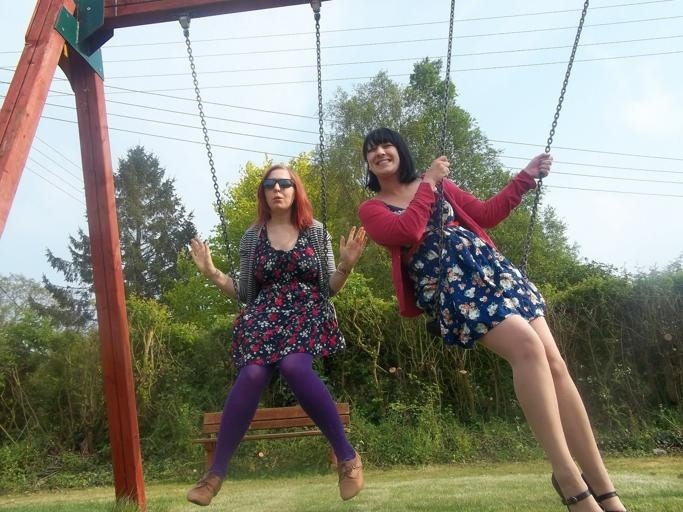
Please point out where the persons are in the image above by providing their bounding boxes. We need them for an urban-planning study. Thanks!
[356,128,626,512]
[188,166,368,507]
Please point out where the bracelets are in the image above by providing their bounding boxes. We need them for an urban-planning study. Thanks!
[335,267,352,277]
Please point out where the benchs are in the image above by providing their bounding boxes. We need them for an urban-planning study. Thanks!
[191,401,352,470]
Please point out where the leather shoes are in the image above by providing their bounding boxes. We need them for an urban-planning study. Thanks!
[186,470,223,506]
[336,450,364,502]
[581,473,628,512]
[551,469,592,512]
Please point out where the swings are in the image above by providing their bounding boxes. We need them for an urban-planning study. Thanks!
[178,0,330,311]
[426,1,589,338]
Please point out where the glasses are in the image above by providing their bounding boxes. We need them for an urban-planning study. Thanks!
[261,178,294,188]
